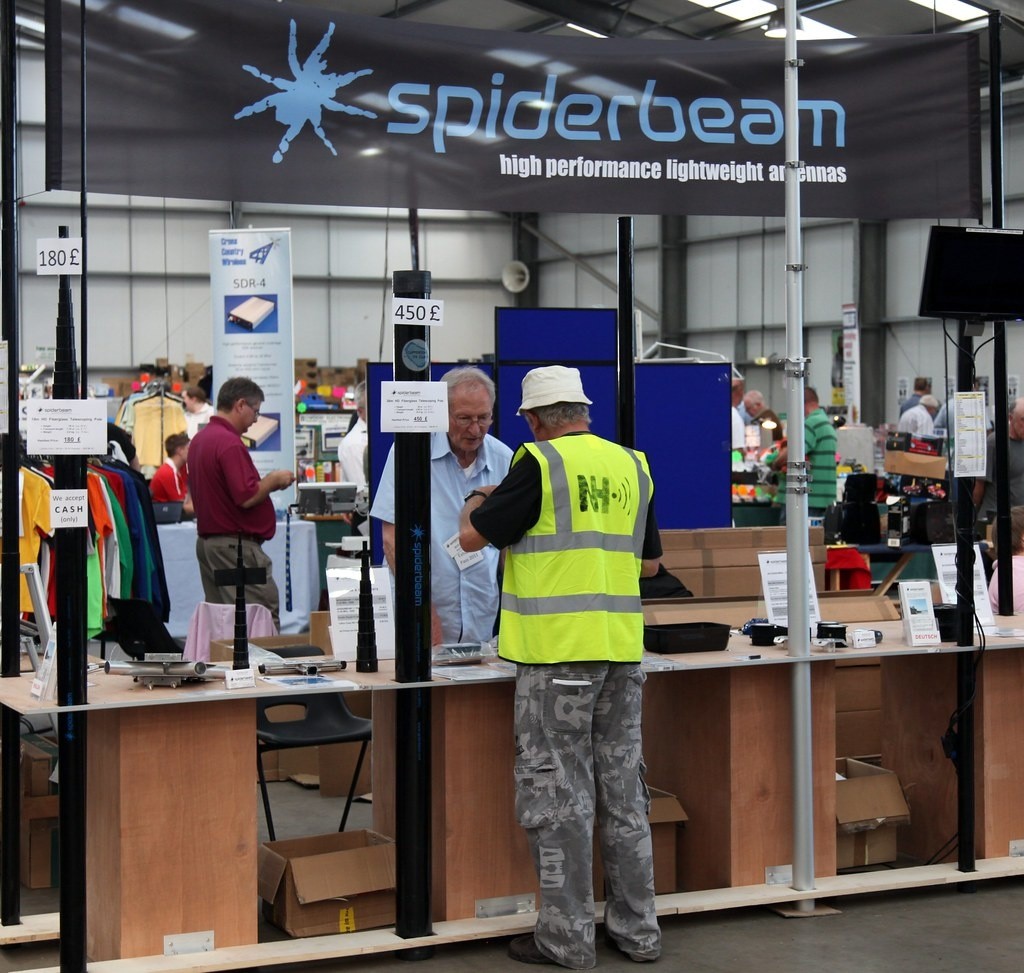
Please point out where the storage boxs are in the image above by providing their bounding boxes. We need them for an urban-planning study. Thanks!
[257,828,396,939]
[171,358,370,397]
[657,526,827,598]
[156,358,168,368]
[20,732,60,890]
[835,757,910,869]
[102,377,135,397]
[592,784,689,902]
[209,632,309,662]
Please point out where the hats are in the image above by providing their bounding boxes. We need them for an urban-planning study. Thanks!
[516,364,593,415]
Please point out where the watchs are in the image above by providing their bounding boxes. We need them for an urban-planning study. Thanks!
[464,490,486,502]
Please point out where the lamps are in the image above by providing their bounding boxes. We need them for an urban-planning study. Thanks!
[764,5,786,39]
[761,414,778,430]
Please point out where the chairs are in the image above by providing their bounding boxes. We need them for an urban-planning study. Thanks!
[254,645,372,842]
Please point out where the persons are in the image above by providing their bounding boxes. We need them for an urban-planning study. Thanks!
[974,396,1024,521]
[107,423,139,471]
[337,380,368,493]
[187,377,296,634]
[744,390,782,440]
[897,378,939,434]
[987,504,1024,613]
[933,399,992,512]
[761,387,837,526]
[149,432,195,522]
[368,367,514,643]
[459,366,663,970]
[732,380,746,448]
[181,385,214,439]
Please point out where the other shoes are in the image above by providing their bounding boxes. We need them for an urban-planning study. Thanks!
[509,935,560,965]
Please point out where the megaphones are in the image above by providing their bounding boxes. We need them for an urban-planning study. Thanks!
[502,260,530,292]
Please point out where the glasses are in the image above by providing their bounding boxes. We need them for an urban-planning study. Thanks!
[238,398,260,418]
[449,409,493,427]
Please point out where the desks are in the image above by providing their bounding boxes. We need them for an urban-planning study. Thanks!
[857,544,932,595]
[0,614,1024,973]
[156,520,321,637]
[824,543,871,591]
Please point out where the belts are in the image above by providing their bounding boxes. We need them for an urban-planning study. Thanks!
[232,534,263,545]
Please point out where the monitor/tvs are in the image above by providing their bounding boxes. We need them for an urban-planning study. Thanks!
[918,226,1024,322]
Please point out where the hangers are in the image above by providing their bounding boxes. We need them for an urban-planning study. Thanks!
[131,377,185,407]
[19,440,116,467]
